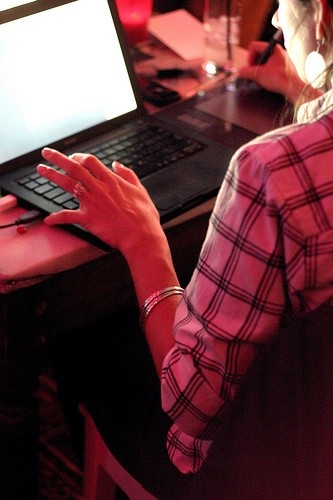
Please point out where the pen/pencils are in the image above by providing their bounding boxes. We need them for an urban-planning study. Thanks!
[244,26,284,89]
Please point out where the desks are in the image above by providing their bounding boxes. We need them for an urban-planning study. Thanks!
[1,37,307,437]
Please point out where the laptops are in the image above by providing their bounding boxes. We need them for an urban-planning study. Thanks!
[0,0,238,252]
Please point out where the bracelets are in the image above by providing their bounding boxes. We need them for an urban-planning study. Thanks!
[141,290,184,328]
[137,285,185,329]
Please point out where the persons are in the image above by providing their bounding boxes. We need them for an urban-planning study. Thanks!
[36,0,332,500]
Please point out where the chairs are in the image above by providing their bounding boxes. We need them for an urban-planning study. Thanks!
[75,301,333,500]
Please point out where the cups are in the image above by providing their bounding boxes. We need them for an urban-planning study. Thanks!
[202,0,244,75]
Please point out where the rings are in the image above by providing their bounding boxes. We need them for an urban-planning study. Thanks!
[72,179,85,199]
